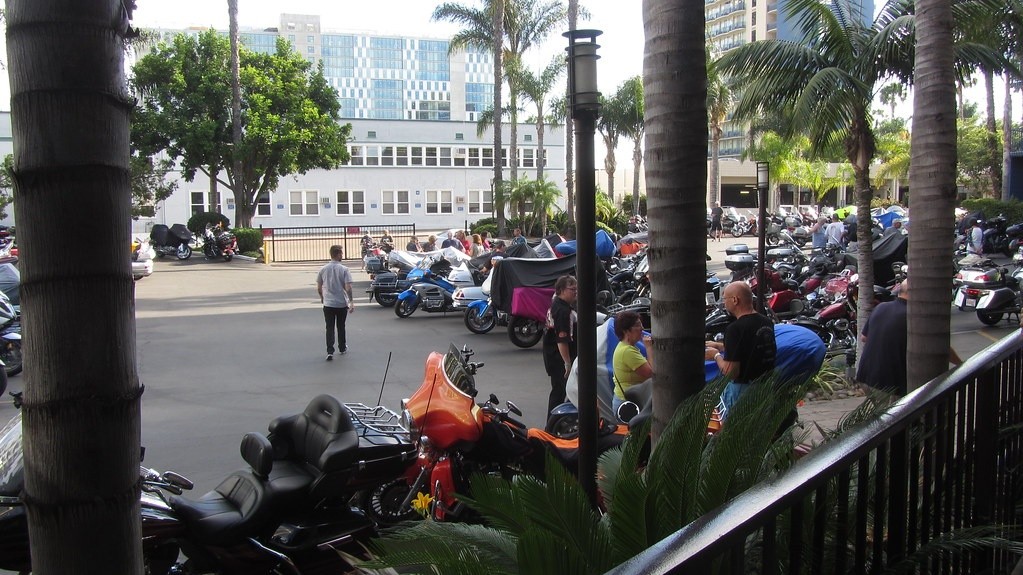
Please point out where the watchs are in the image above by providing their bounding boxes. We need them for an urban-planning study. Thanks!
[712,353,724,360]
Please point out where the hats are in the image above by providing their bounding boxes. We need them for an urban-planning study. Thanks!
[892,219,903,224]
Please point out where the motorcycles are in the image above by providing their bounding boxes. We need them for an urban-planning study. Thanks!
[0,218,22,399]
[363,205,1023,362]
[398,339,727,518]
[132,237,153,281]
[1,387,418,575]
[150,224,192,260]
[200,223,236,261]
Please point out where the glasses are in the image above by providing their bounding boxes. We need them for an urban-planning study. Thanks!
[721,296,733,301]
[564,287,577,292]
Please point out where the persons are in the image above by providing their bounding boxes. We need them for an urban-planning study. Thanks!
[359,228,531,273]
[316,244,354,361]
[542,273,578,435]
[705,279,777,428]
[883,218,902,238]
[856,278,963,446]
[611,310,652,427]
[710,201,725,244]
[964,216,984,257]
[805,211,851,249]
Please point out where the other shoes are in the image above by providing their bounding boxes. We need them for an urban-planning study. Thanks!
[712,239,716,242]
[719,239,720,242]
[339,345,348,354]
[325,354,332,361]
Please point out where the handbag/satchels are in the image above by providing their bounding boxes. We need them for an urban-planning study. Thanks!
[618,401,640,424]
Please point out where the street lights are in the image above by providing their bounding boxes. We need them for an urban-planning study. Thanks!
[753,161,771,314]
[563,29,602,515]
